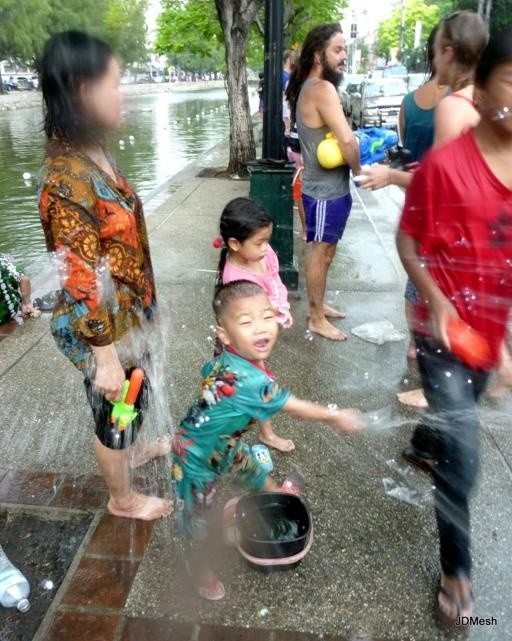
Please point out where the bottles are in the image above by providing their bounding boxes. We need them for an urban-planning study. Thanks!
[0,545,31,614]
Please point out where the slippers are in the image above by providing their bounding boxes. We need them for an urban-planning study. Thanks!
[428,565,480,628]
[184,561,227,601]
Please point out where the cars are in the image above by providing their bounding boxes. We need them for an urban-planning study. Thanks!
[3,72,38,91]
[337,70,431,130]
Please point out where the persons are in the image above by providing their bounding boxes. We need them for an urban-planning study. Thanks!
[1,252,40,327]
[397,28,512,628]
[257,71,264,113]
[393,21,451,360]
[173,277,368,603]
[283,48,290,135]
[359,10,488,410]
[36,31,177,521]
[283,23,367,343]
[213,198,296,455]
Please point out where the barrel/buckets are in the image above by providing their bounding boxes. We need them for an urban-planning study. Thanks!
[235,492,314,572]
